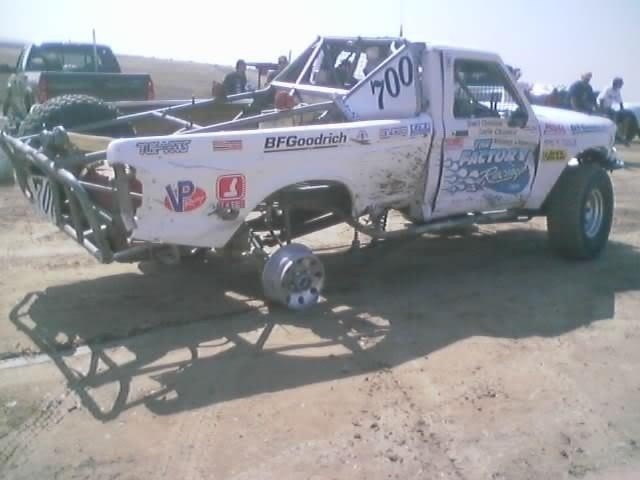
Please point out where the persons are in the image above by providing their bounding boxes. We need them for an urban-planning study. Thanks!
[334,59,357,88]
[597,78,625,117]
[222,59,249,99]
[263,55,289,89]
[569,69,597,114]
